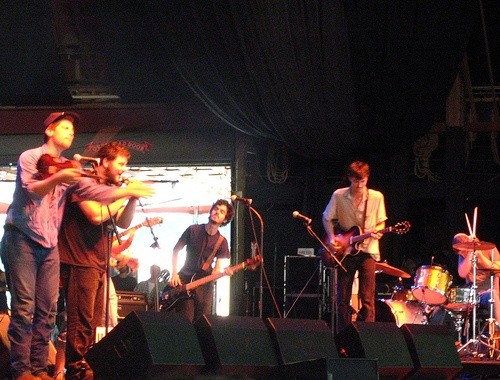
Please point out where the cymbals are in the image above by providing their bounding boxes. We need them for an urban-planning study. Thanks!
[452,241,496,251]
[375,262,411,278]
[476,267,500,274]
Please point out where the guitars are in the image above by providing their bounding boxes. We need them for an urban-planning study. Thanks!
[320,220,410,269]
[112,216,163,242]
[159,254,260,313]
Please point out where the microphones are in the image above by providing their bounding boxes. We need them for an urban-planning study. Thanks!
[74,154,100,164]
[293,211,312,224]
[119,173,134,180]
[230,195,253,204]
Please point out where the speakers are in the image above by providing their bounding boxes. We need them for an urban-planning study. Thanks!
[84,295,465,380]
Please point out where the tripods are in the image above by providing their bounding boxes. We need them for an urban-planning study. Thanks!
[457,242,500,358]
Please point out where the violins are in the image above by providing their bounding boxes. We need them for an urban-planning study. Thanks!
[36,154,123,187]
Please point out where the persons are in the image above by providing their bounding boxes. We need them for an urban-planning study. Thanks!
[1,111,161,380]
[169,200,233,366]
[322,160,388,330]
[452,233,500,326]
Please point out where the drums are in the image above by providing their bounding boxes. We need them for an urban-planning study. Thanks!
[443,287,481,310]
[412,264,454,306]
[355,300,428,327]
[391,286,415,301]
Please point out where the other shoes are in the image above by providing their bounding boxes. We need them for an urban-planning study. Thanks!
[17,373,42,380]
[35,372,55,380]
[80,369,94,380]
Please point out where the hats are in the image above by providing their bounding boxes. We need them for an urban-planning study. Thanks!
[44,111,80,129]
